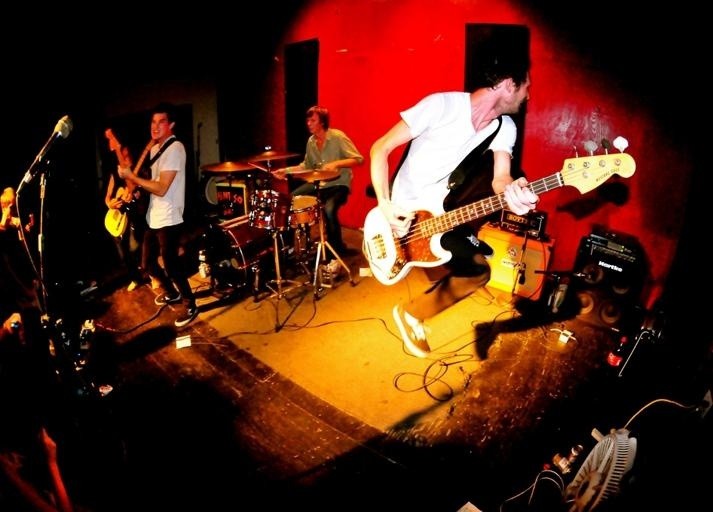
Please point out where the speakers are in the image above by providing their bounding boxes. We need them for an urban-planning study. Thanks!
[20,115,76,185]
[563,229,646,336]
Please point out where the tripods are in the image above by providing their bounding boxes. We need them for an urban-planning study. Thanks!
[309,185,354,301]
[259,233,308,335]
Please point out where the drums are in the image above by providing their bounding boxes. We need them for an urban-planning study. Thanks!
[198,216,273,299]
[249,190,290,232]
[287,196,320,229]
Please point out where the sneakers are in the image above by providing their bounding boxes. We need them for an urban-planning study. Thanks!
[175,305,199,326]
[393,305,431,358]
[152,278,160,289]
[155,292,181,304]
[128,281,137,291]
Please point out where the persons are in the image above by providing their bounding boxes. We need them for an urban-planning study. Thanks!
[269,104,365,270]
[369,55,537,360]
[105,142,149,291]
[116,109,201,329]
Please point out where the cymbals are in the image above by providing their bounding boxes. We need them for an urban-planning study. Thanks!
[199,162,258,172]
[299,169,342,180]
[247,150,300,161]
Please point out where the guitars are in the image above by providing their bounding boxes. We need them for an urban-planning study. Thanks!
[364,136,636,286]
[105,138,156,237]
[105,128,151,243]
